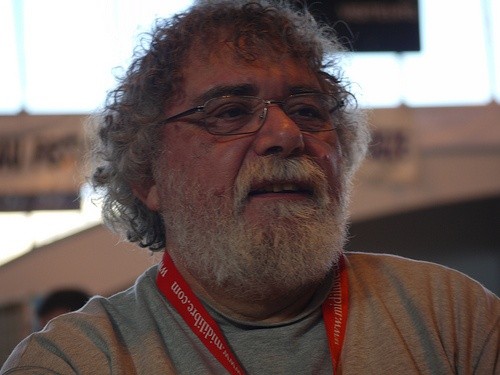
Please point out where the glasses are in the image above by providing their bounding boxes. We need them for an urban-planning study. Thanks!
[155,91,346,137]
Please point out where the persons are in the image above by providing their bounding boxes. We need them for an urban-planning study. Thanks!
[0,0,500,375]
